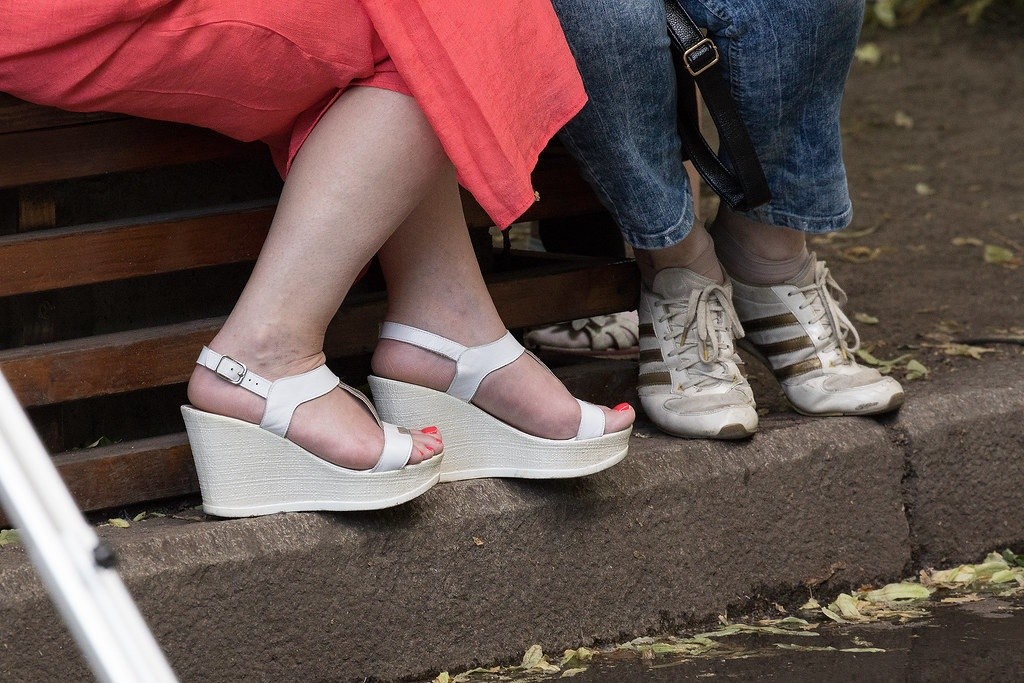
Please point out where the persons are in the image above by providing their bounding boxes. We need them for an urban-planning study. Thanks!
[551,0,905,439]
[0,0,634,519]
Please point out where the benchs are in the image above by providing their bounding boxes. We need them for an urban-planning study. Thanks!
[0,92,639,529]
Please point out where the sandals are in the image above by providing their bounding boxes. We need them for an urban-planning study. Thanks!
[523,314,640,359]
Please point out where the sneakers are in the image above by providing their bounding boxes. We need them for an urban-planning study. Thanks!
[637,264,759,438]
[726,250,905,418]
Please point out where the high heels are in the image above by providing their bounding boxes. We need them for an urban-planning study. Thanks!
[180,344,444,519]
[367,319,635,483]
